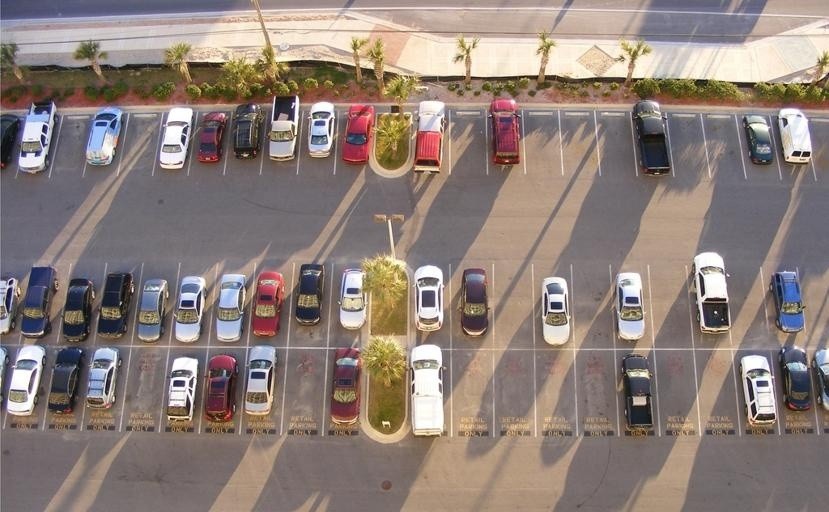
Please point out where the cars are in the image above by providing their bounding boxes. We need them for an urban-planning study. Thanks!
[744,115,773,164]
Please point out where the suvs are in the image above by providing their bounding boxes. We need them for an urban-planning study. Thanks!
[489,98,521,166]
[411,99,447,174]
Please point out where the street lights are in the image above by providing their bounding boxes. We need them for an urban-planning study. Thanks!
[373,212,407,281]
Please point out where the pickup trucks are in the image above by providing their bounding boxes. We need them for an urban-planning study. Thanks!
[631,99,671,176]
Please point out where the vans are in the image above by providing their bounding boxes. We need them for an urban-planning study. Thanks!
[778,108,813,165]
